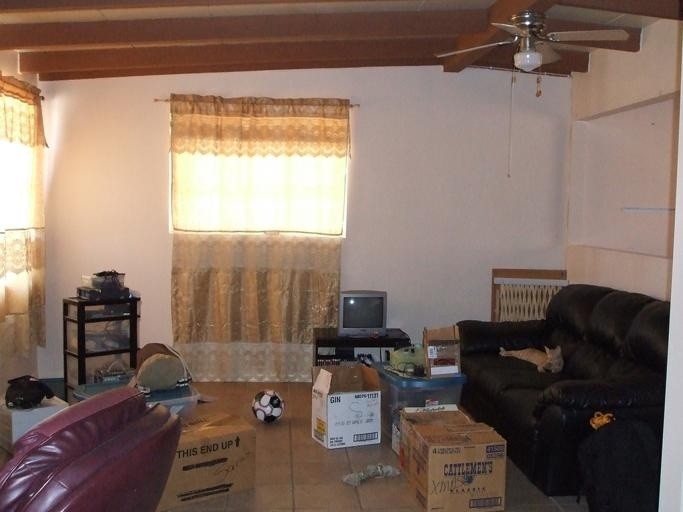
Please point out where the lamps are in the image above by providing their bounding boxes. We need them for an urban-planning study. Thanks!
[513,49,544,98]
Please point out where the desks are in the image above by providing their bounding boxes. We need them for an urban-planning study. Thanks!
[311,326,413,369]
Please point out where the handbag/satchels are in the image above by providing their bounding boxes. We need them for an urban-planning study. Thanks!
[576,416,660,512]
[5,375,54,409]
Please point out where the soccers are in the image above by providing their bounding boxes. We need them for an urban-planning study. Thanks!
[252,389,284,423]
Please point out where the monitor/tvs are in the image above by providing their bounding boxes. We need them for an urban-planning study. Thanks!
[336,290,388,339]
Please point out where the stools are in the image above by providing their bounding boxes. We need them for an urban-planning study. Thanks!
[0,395,69,455]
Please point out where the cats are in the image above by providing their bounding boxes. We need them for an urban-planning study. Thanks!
[498,345,564,373]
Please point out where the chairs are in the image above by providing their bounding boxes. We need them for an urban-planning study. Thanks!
[1,384,184,512]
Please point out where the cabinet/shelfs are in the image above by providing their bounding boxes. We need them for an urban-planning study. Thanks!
[61,293,143,405]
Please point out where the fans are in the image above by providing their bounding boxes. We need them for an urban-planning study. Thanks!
[431,9,632,61]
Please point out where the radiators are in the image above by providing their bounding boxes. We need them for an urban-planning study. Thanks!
[490,274,572,324]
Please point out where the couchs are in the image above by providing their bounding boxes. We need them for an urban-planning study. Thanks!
[455,282,672,500]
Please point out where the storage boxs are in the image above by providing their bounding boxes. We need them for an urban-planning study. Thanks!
[141,384,203,429]
[392,403,508,512]
[153,410,259,511]
[421,322,464,380]
[377,360,469,447]
[309,362,384,452]
